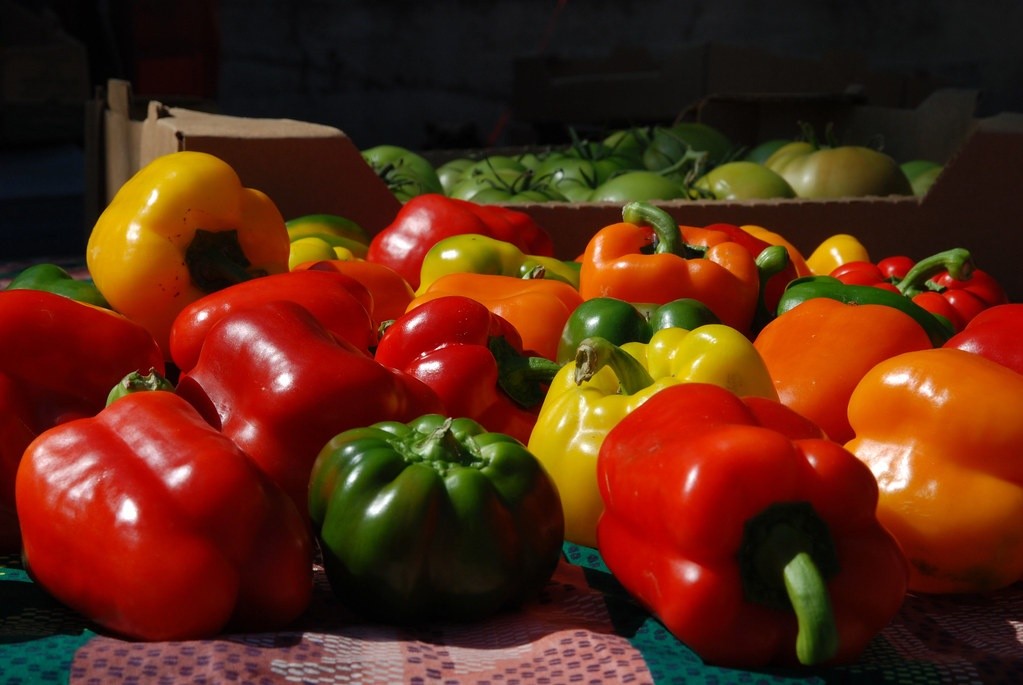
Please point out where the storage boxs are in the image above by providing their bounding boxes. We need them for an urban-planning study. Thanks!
[76,41,1023,301]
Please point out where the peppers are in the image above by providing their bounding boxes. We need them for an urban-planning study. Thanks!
[0,149,1023,665]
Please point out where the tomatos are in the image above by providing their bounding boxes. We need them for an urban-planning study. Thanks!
[359,122,951,202]
